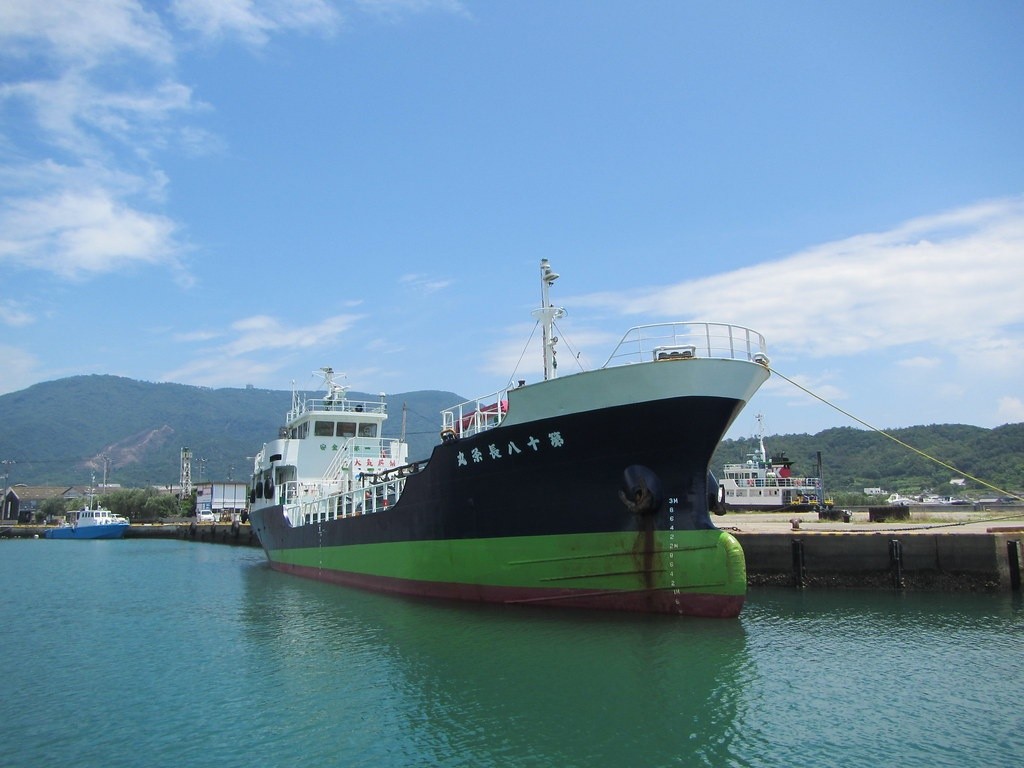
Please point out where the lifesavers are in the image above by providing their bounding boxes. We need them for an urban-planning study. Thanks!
[241,509,247,524]
[255,482,263,498]
[356,405,362,412]
[264,479,273,499]
[250,489,255,503]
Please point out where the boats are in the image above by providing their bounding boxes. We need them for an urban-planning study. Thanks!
[45,474,130,539]
[718,413,834,512]
[247,258,771,620]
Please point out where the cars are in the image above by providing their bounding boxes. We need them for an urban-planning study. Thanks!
[196,510,215,522]
